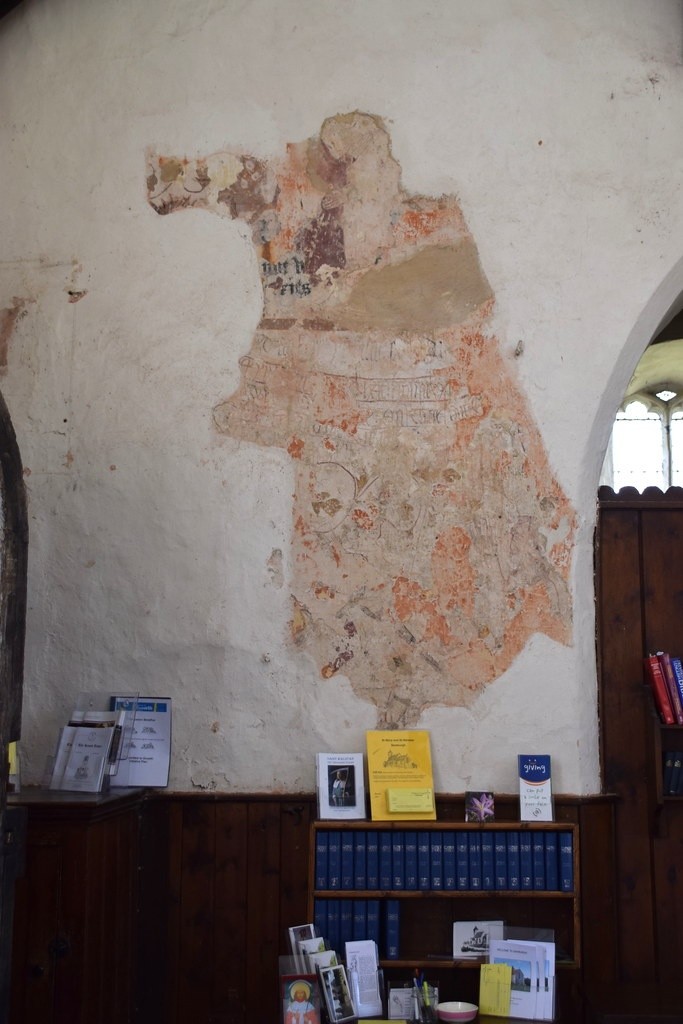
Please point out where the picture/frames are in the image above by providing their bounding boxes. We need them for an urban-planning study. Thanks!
[280,973,320,1024]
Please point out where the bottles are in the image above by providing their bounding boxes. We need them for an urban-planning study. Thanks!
[411,985,438,1024]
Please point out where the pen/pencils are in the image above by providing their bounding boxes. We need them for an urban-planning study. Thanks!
[413,970,433,1024]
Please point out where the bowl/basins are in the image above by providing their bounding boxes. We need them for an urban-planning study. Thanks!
[437,1001,478,1024]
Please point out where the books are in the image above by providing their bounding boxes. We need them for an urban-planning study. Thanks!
[366,900,380,942]
[353,901,367,942]
[315,753,365,818]
[386,900,399,960]
[313,898,355,964]
[49,693,174,792]
[366,730,437,820]
[315,830,573,893]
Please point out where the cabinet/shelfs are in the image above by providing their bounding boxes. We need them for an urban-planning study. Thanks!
[310,822,582,982]
